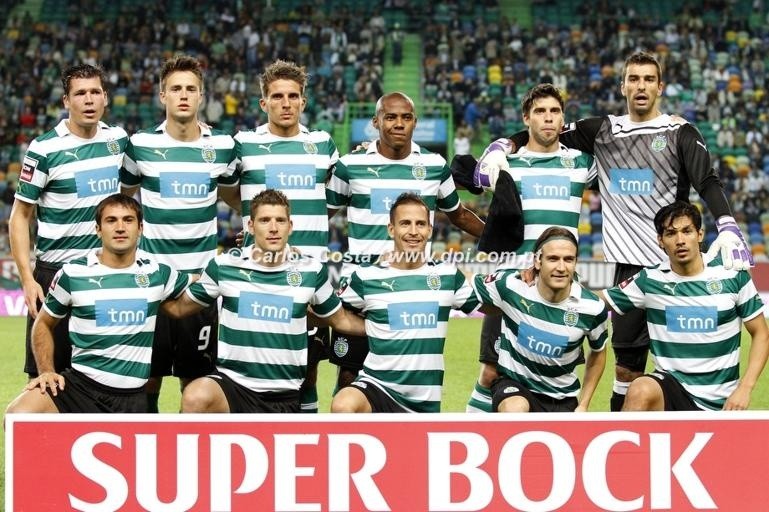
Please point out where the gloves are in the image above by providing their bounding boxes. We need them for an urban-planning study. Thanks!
[707,215,755,270]
[473,138,513,193]
